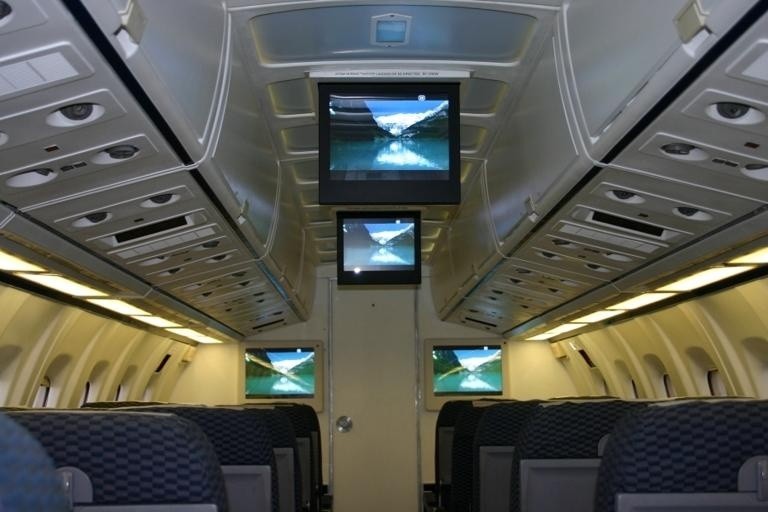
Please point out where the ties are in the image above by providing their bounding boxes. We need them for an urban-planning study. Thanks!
[318,81,461,205]
[424,336,510,411]
[336,210,422,285]
[237,339,325,413]
